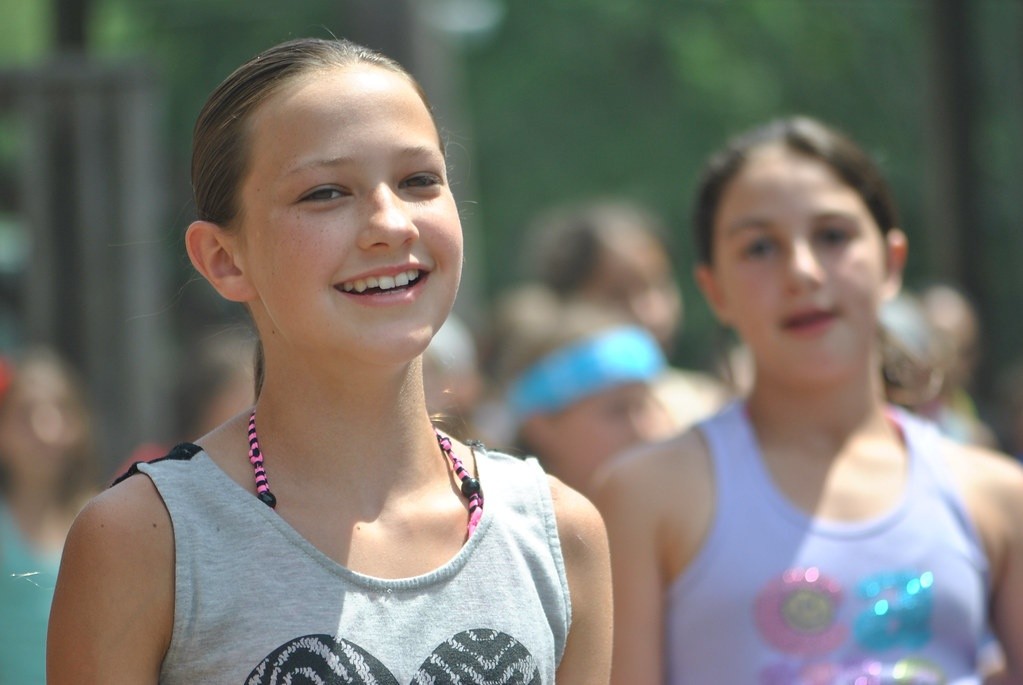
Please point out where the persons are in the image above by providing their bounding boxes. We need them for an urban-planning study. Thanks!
[0,348,106,685]
[480,202,724,501]
[584,113,1022,685]
[47,38,613,685]
[114,324,253,482]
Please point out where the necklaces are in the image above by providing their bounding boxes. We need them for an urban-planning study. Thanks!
[237,408,482,545]
